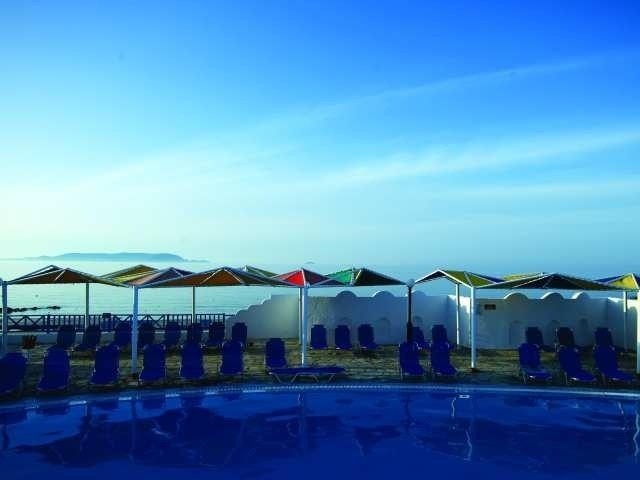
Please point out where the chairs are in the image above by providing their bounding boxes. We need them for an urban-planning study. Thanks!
[0,319,293,398]
[516,324,635,388]
[309,320,458,382]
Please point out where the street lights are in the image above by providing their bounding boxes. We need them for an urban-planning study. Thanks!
[406,278,415,342]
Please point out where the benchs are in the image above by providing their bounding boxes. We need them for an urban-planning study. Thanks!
[266,366,344,385]
